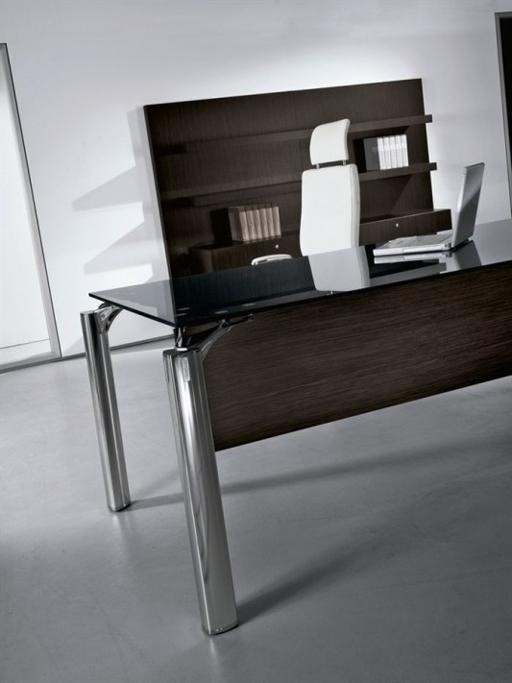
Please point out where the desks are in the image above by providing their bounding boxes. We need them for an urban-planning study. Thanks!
[80,218,512,635]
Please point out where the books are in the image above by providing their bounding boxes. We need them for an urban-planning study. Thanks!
[364,134,409,171]
[228,203,283,242]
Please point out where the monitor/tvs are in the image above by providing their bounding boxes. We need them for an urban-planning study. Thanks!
[373,162,484,257]
[373,240,482,275]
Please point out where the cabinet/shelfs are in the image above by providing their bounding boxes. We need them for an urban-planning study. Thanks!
[142,78,451,274]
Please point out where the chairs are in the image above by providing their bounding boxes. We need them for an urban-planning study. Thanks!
[251,119,360,265]
